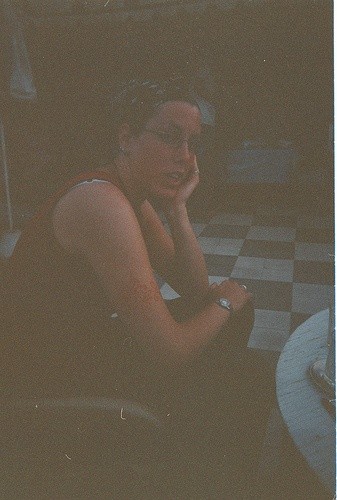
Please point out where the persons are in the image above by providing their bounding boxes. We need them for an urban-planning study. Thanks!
[0,77,255,403]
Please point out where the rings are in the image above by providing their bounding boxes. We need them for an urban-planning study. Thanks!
[191,169,200,175]
[240,284,247,291]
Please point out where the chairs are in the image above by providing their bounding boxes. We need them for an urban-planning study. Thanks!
[2,228,174,434]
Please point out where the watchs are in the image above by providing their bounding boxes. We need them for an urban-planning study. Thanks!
[214,297,234,312]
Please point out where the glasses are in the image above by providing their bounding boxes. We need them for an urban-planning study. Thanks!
[140,127,207,156]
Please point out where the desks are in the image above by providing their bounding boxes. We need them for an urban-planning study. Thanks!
[276,307,336,496]
